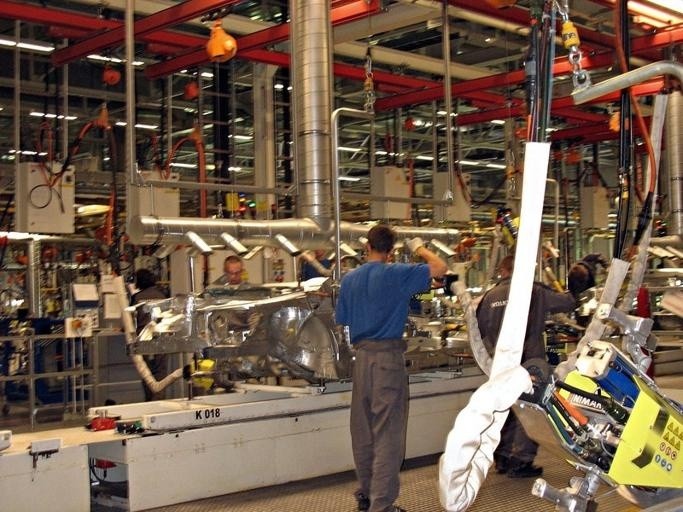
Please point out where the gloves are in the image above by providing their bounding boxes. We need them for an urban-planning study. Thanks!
[405,237,424,254]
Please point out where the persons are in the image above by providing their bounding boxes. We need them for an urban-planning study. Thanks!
[302,250,330,286]
[130,268,189,400]
[215,256,248,288]
[569,254,612,325]
[335,224,449,512]
[478,255,578,480]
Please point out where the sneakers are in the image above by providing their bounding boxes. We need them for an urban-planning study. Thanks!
[358,492,407,511]
[495,461,543,477]
